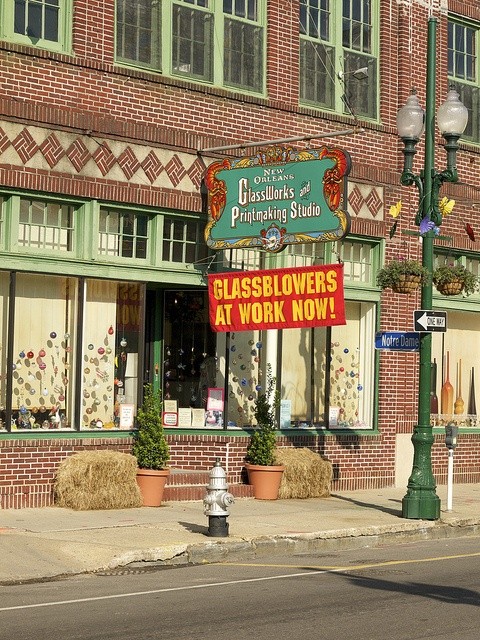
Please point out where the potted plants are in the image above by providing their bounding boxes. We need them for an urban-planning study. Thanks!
[375,256,431,297]
[131,379,172,510]
[433,263,480,298]
[243,396,286,501]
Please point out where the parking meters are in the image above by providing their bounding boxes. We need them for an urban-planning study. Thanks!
[445,421,458,450]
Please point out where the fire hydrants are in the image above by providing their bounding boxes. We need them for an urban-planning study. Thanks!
[203,457,236,537]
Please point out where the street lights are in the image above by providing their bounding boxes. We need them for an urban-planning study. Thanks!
[397,14,467,523]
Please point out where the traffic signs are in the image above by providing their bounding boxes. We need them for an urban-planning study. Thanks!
[413,310,447,333]
[374,331,420,352]
